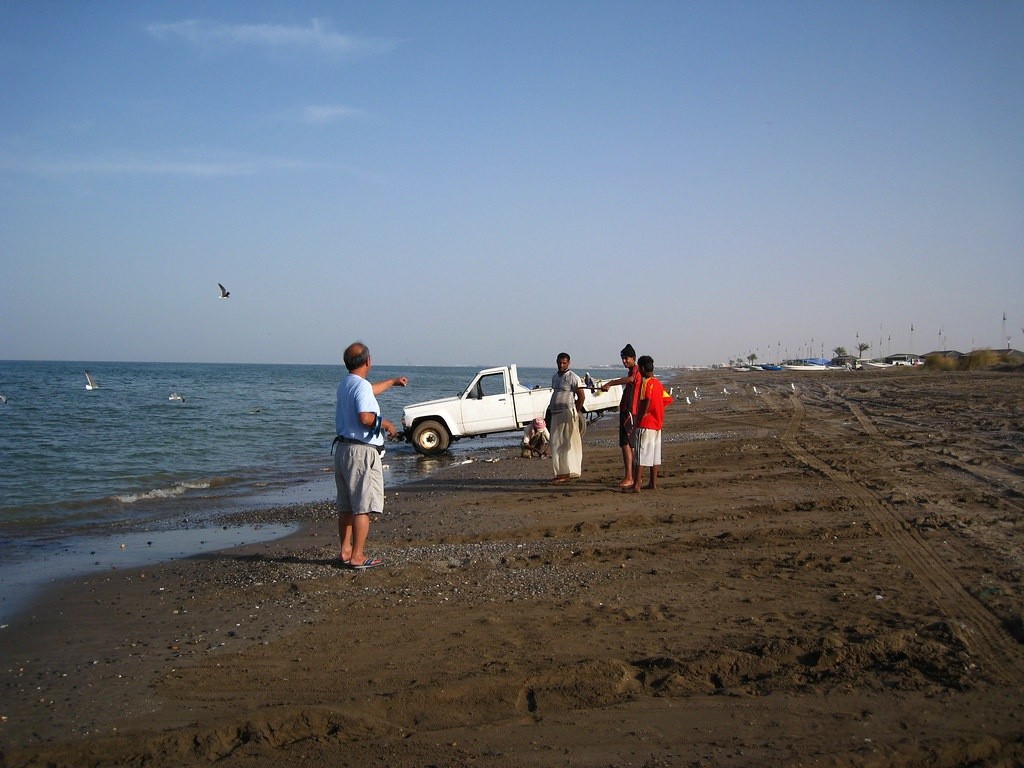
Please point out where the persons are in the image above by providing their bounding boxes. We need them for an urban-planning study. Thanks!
[601,344,673,493]
[550,352,585,482]
[520,417,552,458]
[334,343,409,569]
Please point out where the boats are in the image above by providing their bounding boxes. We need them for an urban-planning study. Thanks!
[733,363,783,372]
[169,391,181,401]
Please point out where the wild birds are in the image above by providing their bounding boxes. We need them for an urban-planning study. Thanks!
[251,408,261,413]
[790,383,796,391]
[0,395,8,403]
[753,387,761,395]
[720,387,738,396]
[168,392,186,403]
[668,385,702,407]
[217,282,231,300]
[83,368,103,390]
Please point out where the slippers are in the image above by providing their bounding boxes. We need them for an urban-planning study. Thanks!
[337,557,351,564]
[349,558,385,569]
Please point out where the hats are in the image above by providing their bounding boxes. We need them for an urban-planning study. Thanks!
[533,418,546,428]
[621,344,636,357]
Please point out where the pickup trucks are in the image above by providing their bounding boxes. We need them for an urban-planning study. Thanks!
[401,364,624,457]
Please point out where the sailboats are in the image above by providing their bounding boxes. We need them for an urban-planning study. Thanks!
[84,370,99,391]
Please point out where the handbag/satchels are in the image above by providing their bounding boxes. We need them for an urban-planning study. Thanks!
[331,436,386,459]
